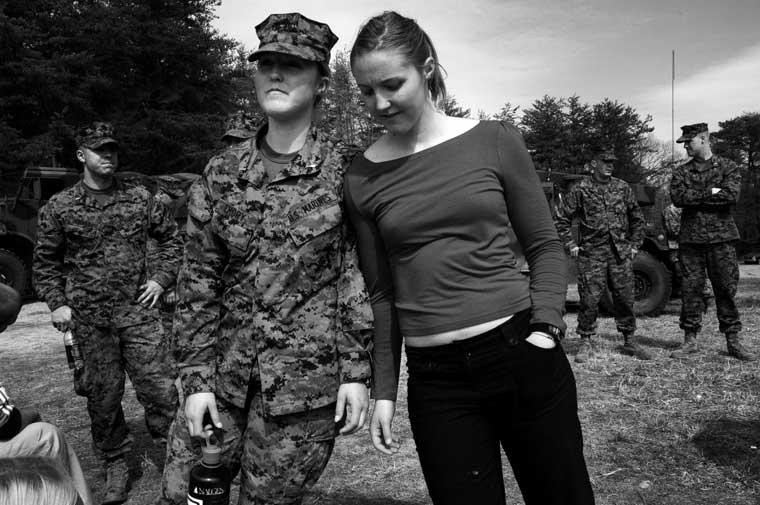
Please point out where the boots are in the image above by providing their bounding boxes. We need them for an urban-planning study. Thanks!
[620,329,653,361]
[671,328,699,359]
[574,334,594,364]
[726,331,757,363]
[99,455,137,501]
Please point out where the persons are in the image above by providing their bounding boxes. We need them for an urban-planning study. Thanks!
[33,122,182,503]
[343,11,595,504]
[670,123,758,362]
[553,151,654,361]
[220,113,265,147]
[0,248,41,442]
[159,11,375,503]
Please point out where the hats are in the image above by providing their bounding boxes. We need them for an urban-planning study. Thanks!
[158,175,186,197]
[73,121,119,150]
[246,11,340,63]
[677,121,709,142]
[593,144,618,160]
[221,114,260,140]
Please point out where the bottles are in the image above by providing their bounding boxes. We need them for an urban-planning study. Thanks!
[187,432,229,505]
[62,328,85,371]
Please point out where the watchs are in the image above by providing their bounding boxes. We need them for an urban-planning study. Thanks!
[532,323,565,344]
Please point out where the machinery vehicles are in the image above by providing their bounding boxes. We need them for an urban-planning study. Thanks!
[526,147,687,318]
[0,164,89,303]
